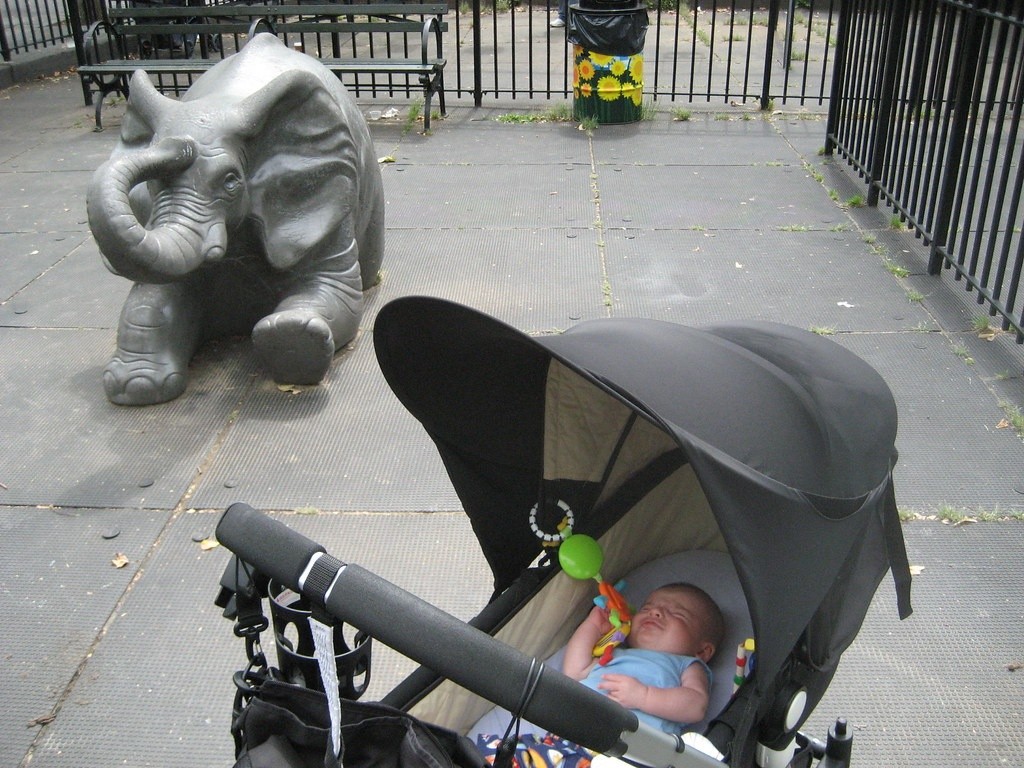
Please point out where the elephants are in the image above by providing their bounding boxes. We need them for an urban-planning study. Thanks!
[85,32,386,407]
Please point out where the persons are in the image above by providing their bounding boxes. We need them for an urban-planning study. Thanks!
[550,0,579,27]
[477,580,724,768]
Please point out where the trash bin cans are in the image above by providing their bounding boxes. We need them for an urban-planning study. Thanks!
[568,0,651,127]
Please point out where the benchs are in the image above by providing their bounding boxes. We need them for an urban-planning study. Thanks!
[75,3,449,132]
